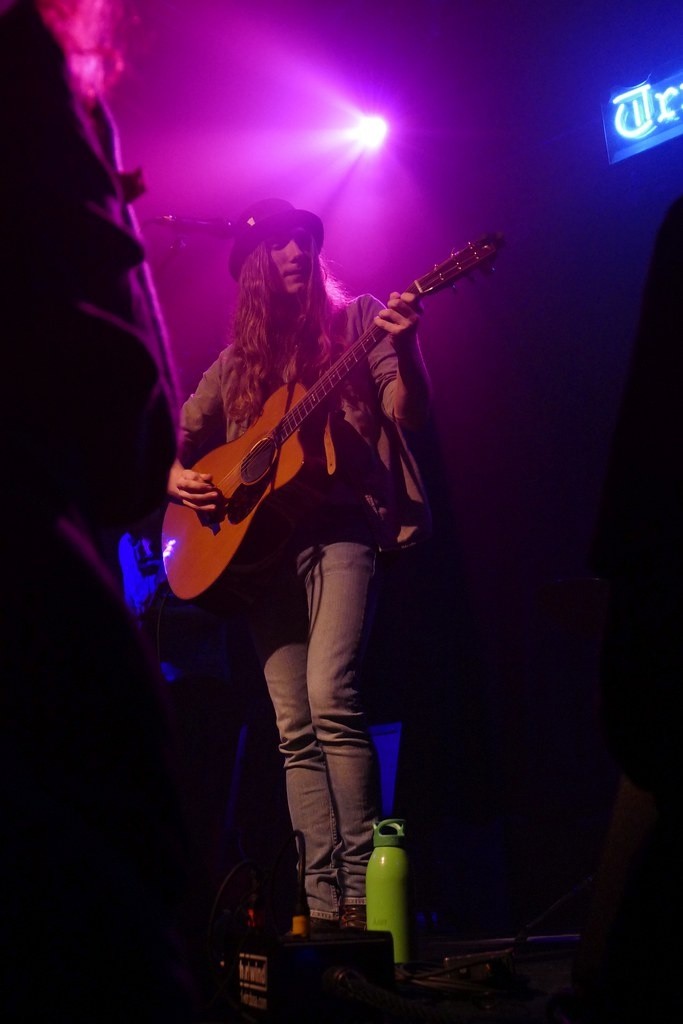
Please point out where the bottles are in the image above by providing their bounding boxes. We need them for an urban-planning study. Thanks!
[366,818,409,964]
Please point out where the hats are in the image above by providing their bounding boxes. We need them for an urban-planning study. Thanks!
[229,197,324,279]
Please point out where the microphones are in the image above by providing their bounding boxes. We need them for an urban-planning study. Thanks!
[160,216,233,239]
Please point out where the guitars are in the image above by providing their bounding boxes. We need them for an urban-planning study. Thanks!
[159,230,507,609]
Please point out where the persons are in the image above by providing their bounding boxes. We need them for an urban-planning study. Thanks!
[560,197,683,1024]
[0,0,179,1024]
[166,198,436,930]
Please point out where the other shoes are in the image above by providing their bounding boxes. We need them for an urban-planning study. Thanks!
[308,905,366,933]
